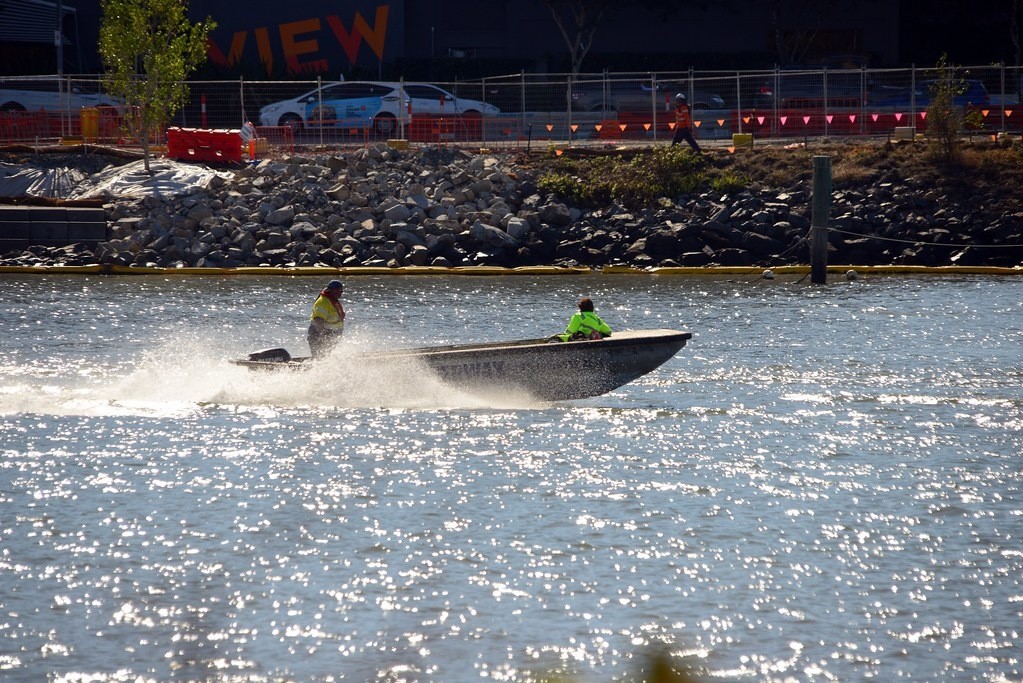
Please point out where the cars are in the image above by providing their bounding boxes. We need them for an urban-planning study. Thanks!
[259,80,414,136]
[873,78,997,111]
[574,82,727,137]
[0,76,130,127]
[390,82,500,133]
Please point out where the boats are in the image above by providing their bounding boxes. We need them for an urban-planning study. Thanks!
[194,327,693,411]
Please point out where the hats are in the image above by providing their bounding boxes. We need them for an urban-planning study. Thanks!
[326,280,343,289]
[580,297,593,309]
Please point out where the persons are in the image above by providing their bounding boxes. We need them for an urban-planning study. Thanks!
[307,280,346,362]
[671,92,702,155]
[543,297,612,343]
